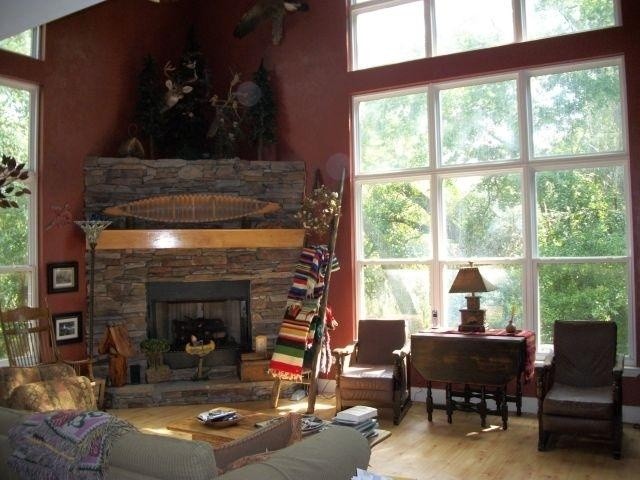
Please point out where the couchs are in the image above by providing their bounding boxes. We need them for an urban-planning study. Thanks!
[0,363,370,480]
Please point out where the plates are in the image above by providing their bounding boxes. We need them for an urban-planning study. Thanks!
[195,412,246,428]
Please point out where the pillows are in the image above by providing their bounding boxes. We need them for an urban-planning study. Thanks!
[0,363,76,409]
[8,376,98,413]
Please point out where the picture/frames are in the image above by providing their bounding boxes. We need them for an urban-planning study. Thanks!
[50,312,83,346]
[46,261,79,294]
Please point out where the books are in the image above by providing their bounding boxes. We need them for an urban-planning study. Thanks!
[329,403,380,441]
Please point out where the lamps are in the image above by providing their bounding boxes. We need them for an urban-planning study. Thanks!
[448,261,497,332]
[73,221,112,382]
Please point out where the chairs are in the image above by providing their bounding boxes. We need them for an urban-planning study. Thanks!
[536,319,622,460]
[0,305,93,378]
[332,319,412,425]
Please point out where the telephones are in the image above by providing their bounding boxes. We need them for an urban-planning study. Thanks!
[430,309,440,330]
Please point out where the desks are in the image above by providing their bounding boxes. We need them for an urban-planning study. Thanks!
[407,328,537,430]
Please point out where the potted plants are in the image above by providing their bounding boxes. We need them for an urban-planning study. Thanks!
[140,338,170,370]
[506,300,519,333]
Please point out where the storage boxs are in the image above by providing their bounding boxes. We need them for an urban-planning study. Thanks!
[237,354,276,382]
